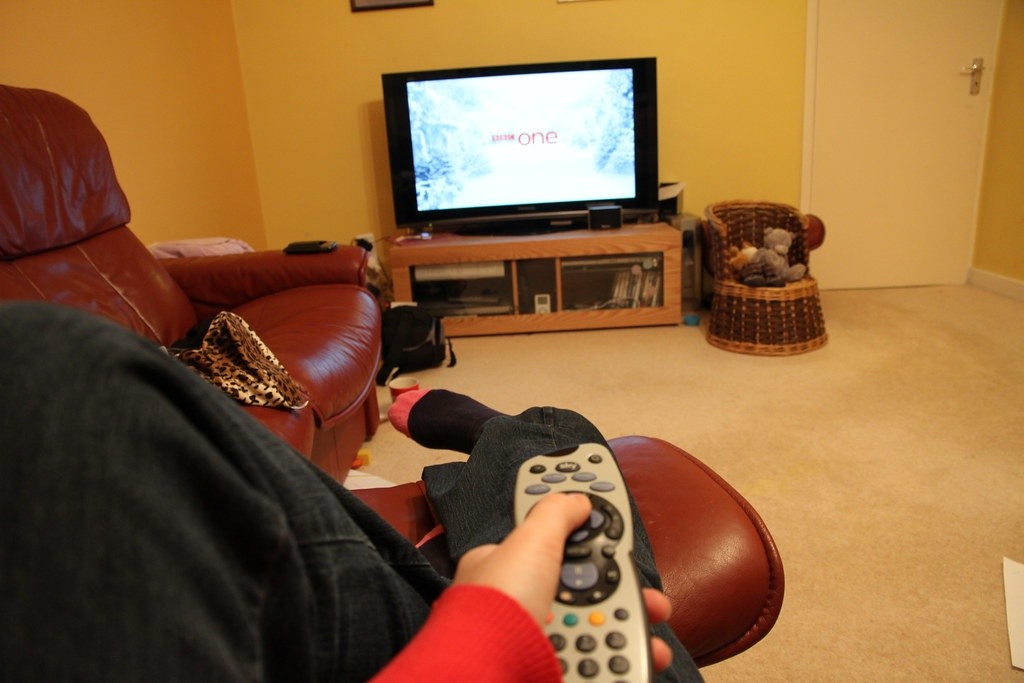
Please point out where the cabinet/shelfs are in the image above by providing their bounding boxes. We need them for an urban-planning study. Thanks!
[389,222,682,337]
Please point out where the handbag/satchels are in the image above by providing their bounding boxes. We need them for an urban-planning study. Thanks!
[377,302,456,386]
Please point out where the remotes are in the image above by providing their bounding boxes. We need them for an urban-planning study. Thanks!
[511,442,653,683]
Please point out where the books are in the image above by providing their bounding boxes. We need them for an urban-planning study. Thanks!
[609,271,662,307]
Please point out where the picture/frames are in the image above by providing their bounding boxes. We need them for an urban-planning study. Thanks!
[350,0,435,13]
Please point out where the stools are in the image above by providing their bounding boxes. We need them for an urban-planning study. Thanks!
[671,213,703,313]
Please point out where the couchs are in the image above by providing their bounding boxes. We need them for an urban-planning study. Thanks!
[347,436,786,670]
[0,84,382,488]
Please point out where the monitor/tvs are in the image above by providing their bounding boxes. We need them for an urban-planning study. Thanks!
[378,57,661,235]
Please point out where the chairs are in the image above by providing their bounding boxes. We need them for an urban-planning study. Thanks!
[705,199,828,356]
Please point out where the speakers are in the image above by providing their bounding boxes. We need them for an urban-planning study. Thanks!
[585,204,624,233]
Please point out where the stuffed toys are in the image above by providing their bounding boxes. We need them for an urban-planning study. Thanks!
[723,226,806,286]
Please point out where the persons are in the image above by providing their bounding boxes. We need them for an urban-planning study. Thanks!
[1,301,706,682]
